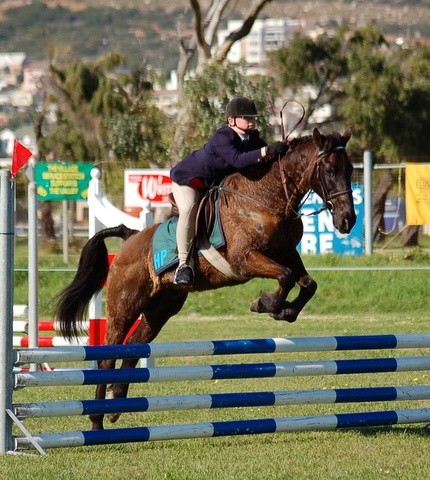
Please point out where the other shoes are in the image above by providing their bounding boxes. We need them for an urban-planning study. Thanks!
[175,263,193,284]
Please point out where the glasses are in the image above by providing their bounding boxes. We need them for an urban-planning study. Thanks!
[241,116,258,121]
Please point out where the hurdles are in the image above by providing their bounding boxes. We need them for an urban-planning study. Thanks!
[0,166,429,454]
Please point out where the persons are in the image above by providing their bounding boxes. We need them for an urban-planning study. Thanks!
[170,97,285,286]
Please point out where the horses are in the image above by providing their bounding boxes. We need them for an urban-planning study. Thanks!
[45,125,357,431]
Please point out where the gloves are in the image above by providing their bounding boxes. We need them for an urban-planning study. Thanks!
[267,141,289,156]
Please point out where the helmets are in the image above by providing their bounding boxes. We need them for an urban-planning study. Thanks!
[228,97,261,117]
